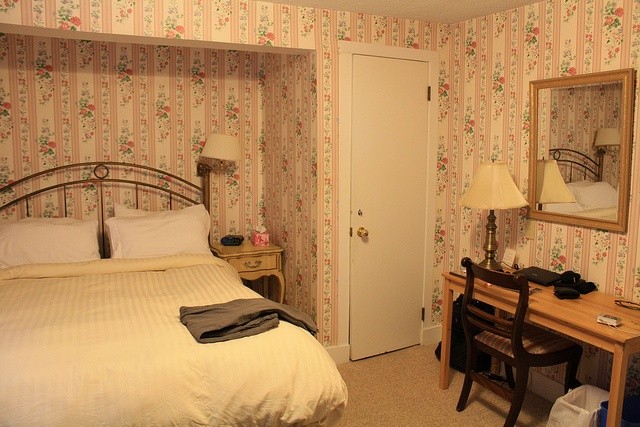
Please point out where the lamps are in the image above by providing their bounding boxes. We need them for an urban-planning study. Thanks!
[199,132,241,163]
[593,126,620,160]
[535,159,575,212]
[459,162,529,270]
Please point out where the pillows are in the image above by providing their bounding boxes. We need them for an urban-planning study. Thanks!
[114,204,211,237]
[542,202,584,212]
[568,180,617,209]
[0,217,101,267]
[104,202,211,258]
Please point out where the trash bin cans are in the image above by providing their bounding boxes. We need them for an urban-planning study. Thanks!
[559,384,609,427]
[600,394,640,427]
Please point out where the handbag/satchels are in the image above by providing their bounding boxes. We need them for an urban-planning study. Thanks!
[435,293,495,373]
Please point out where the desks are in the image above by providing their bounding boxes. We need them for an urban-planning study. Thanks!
[439,264,640,426]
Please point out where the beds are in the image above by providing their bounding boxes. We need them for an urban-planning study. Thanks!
[0,160,348,425]
[548,146,619,220]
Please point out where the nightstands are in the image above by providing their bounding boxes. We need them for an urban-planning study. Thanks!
[210,238,285,304]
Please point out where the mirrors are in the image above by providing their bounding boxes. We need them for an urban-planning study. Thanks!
[527,66,635,232]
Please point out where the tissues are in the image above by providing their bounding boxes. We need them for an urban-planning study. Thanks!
[251,224,269,247]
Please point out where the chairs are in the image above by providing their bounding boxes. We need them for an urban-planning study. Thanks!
[456,255,583,426]
